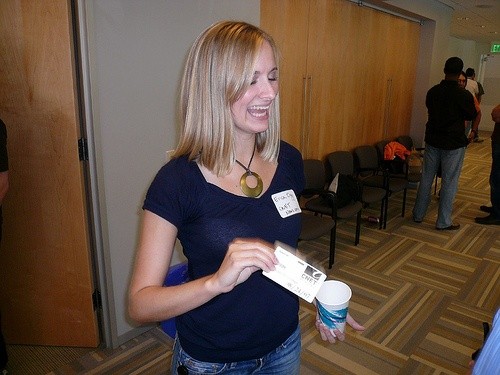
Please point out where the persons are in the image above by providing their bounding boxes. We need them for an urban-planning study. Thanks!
[411,57,500,231]
[0,118,10,207]
[127,20,367,375]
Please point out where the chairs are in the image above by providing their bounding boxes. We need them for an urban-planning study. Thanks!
[297,136,438,269]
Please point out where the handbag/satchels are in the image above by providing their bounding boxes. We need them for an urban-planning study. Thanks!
[327,173,360,196]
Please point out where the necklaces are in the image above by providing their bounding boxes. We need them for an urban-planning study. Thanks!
[236,139,263,198]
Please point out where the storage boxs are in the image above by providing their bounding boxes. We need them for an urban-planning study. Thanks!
[161,261,189,339]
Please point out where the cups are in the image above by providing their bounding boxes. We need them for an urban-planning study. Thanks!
[315,279,352,337]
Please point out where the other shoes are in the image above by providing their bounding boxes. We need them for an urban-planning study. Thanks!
[475,214,500,225]
[479,206,493,213]
[412,219,421,223]
[436,224,460,230]
